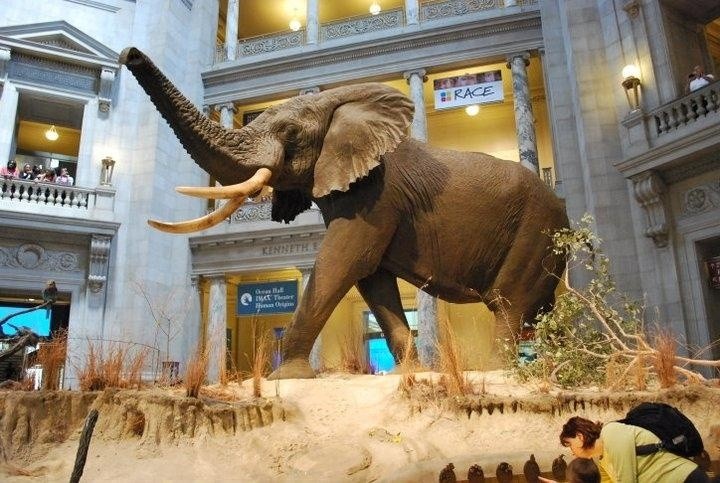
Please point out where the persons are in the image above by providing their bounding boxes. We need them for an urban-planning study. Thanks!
[1,159,74,205]
[564,457,601,483]
[683,65,715,117]
[538,416,710,483]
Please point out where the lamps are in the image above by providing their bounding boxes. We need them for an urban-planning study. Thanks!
[290,7,301,31]
[618,65,641,110]
[465,105,481,116]
[369,1,380,14]
[44,125,60,141]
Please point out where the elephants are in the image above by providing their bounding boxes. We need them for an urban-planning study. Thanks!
[117,46,596,382]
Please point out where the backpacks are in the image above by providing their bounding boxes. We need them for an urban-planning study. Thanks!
[604,402,704,458]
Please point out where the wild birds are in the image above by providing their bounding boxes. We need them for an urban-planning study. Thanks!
[41,280,58,320]
